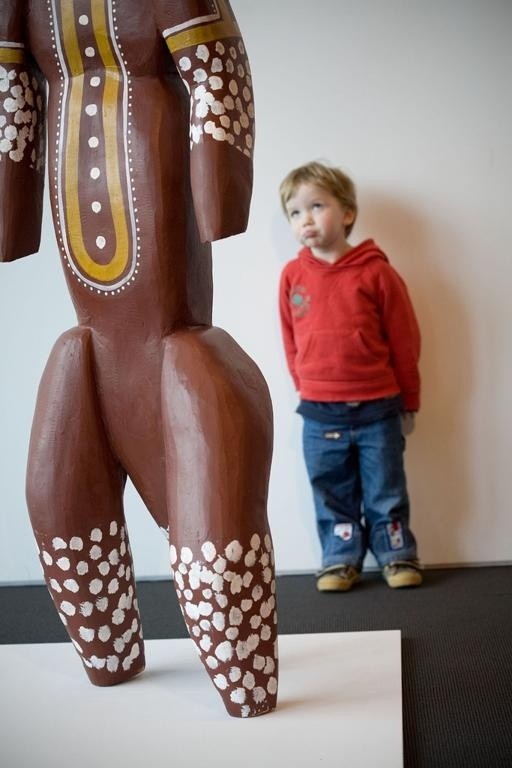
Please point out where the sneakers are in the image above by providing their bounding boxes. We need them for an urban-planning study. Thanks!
[316,565,363,592]
[383,559,424,588]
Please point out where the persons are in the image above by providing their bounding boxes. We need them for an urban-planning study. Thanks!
[276,159,425,592]
